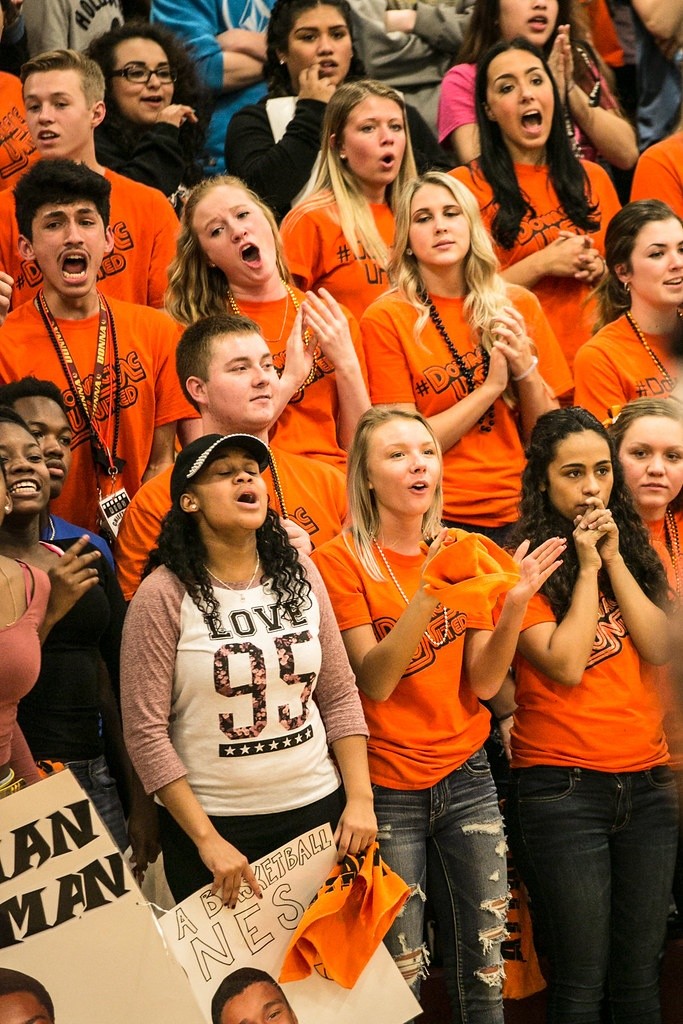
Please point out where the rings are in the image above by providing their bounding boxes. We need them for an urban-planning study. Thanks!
[515,330,522,335]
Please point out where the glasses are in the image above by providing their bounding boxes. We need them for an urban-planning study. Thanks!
[111,66,178,84]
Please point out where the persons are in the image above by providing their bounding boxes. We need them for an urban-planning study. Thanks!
[211,967,299,1024]
[610,397,683,776]
[121,432,378,910]
[116,314,351,601]
[0,967,55,1024]
[0,0,683,550]
[309,405,569,1024]
[491,406,683,1024]
[0,375,162,873]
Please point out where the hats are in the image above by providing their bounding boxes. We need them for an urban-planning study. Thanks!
[169,433,271,504]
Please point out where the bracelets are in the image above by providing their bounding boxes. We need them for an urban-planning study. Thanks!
[512,356,537,380]
[597,254,605,278]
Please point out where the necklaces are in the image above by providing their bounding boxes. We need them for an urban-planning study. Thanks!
[417,285,495,432]
[626,311,675,389]
[49,517,56,540]
[202,549,259,599]
[227,278,317,393]
[36,287,120,459]
[268,446,289,520]
[665,510,683,604]
[371,528,448,645]
[0,569,18,627]
